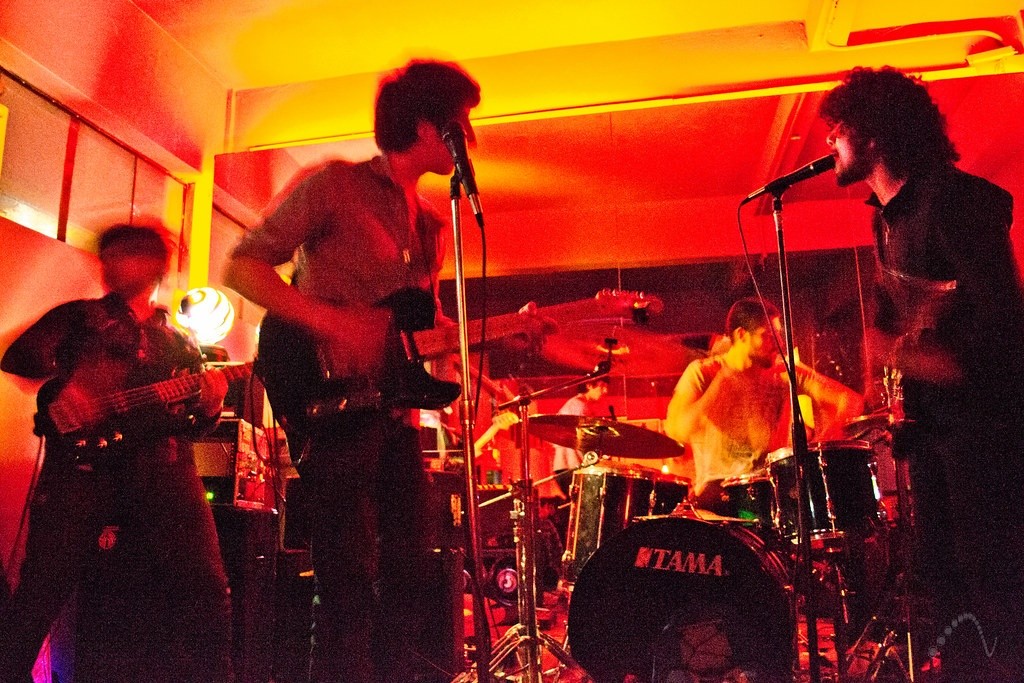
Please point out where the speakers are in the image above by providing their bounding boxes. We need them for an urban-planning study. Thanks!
[52,504,279,683]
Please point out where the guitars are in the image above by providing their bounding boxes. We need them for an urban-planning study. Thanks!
[254,288,666,443]
[32,349,259,464]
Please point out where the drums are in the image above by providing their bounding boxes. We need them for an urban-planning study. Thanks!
[772,447,887,533]
[567,515,803,682]
[708,471,798,523]
[559,469,694,562]
[477,481,543,548]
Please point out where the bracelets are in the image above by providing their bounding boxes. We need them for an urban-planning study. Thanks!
[892,335,909,369]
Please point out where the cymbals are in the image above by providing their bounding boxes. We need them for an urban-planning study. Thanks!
[525,411,682,459]
[537,318,692,378]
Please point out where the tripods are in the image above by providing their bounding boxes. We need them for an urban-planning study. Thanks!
[843,446,938,683]
[453,352,615,683]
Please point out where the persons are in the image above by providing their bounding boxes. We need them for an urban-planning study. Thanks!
[221,58,562,683]
[553,377,610,495]
[665,299,866,497]
[826,68,1024,683]
[0,224,232,683]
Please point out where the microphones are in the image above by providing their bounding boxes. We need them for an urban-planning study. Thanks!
[440,119,483,227]
[741,154,835,206]
